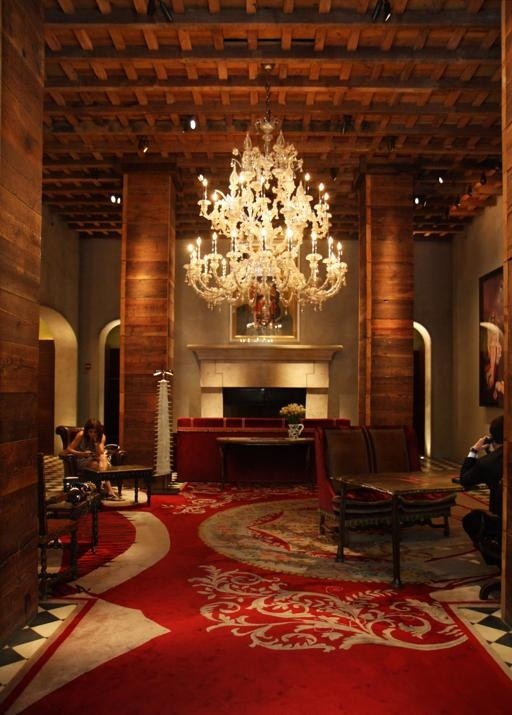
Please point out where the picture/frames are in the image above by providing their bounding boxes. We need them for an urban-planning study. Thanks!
[229,239,301,343]
[479,266,504,407]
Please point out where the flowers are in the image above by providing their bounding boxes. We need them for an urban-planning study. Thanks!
[280,404,306,423]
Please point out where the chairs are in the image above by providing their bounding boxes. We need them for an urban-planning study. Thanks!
[362,425,458,542]
[37,453,101,550]
[38,532,92,602]
[56,426,128,480]
[314,425,403,537]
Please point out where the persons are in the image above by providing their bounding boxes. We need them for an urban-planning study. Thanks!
[66,418,124,501]
[459,413,505,574]
[243,286,292,334]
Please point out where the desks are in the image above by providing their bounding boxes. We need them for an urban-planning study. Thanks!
[329,471,490,585]
[174,418,350,482]
[216,436,315,490]
[79,465,153,506]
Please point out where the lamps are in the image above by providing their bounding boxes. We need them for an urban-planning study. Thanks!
[182,64,348,329]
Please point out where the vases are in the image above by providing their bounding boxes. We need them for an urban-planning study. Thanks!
[288,424,304,438]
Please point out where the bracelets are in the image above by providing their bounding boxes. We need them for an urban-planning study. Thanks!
[470,447,478,454]
